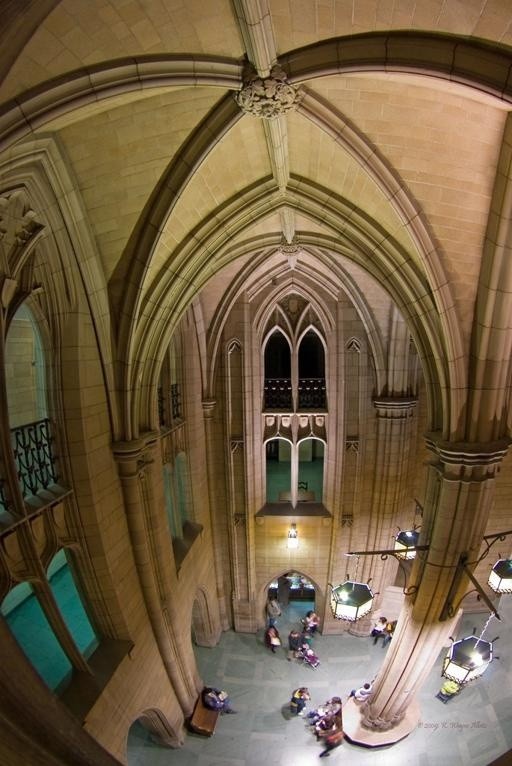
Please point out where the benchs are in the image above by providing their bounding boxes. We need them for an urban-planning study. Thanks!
[189,695,221,737]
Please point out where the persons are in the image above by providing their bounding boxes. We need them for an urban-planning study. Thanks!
[264,622,283,655]
[313,714,337,739]
[203,685,236,714]
[302,641,319,667]
[371,616,387,645]
[267,596,283,626]
[290,687,311,717]
[302,696,342,728]
[287,630,310,662]
[381,620,398,647]
[301,609,321,639]
[349,682,372,703]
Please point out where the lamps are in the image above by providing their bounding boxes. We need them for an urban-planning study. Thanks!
[390,521,423,561]
[488,556,512,595]
[325,555,380,623]
[440,611,495,685]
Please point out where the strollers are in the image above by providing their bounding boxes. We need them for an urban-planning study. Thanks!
[298,642,322,671]
